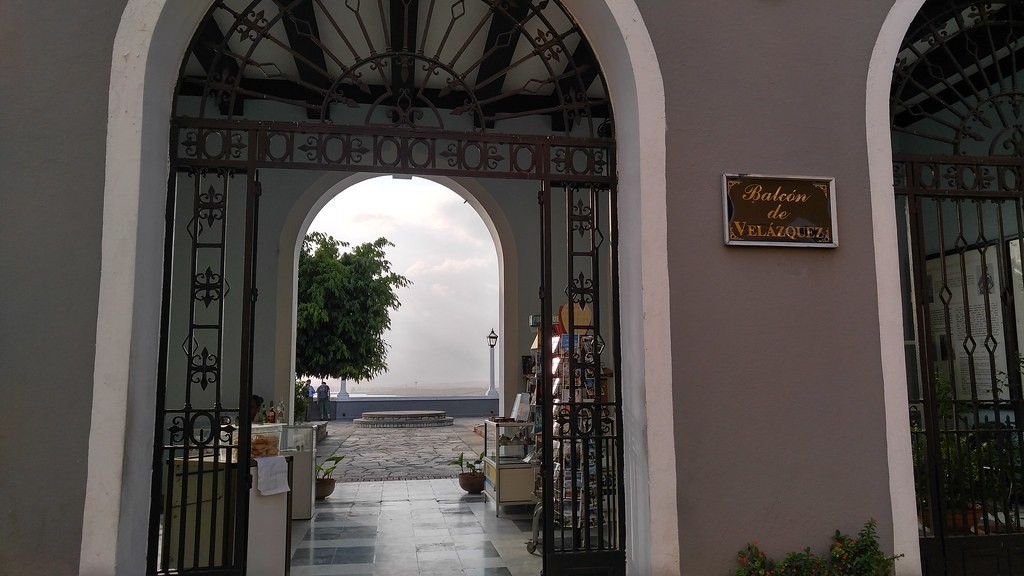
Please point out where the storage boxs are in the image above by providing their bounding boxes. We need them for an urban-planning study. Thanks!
[215,422,289,463]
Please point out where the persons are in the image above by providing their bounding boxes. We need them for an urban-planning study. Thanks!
[316,382,332,421]
[305,379,315,422]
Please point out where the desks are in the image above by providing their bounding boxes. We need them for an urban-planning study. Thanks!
[159,451,254,574]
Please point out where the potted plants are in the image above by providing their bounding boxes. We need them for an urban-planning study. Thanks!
[315,455,347,500]
[447,450,485,495]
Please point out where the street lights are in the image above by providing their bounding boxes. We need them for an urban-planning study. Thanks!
[486,328,498,396]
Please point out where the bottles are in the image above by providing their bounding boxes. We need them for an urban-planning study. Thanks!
[220,425,230,461]
[268,401,276,423]
[276,403,284,423]
[262,404,267,425]
[281,401,286,422]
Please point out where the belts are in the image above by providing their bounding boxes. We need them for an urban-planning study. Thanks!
[309,397,312,398]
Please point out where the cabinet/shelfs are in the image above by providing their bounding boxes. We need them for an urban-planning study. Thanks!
[483,419,539,518]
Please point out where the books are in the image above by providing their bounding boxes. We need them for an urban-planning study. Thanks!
[510,333,612,529]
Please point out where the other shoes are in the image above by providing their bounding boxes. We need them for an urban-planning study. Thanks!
[328,417,331,421]
[320,419,324,421]
